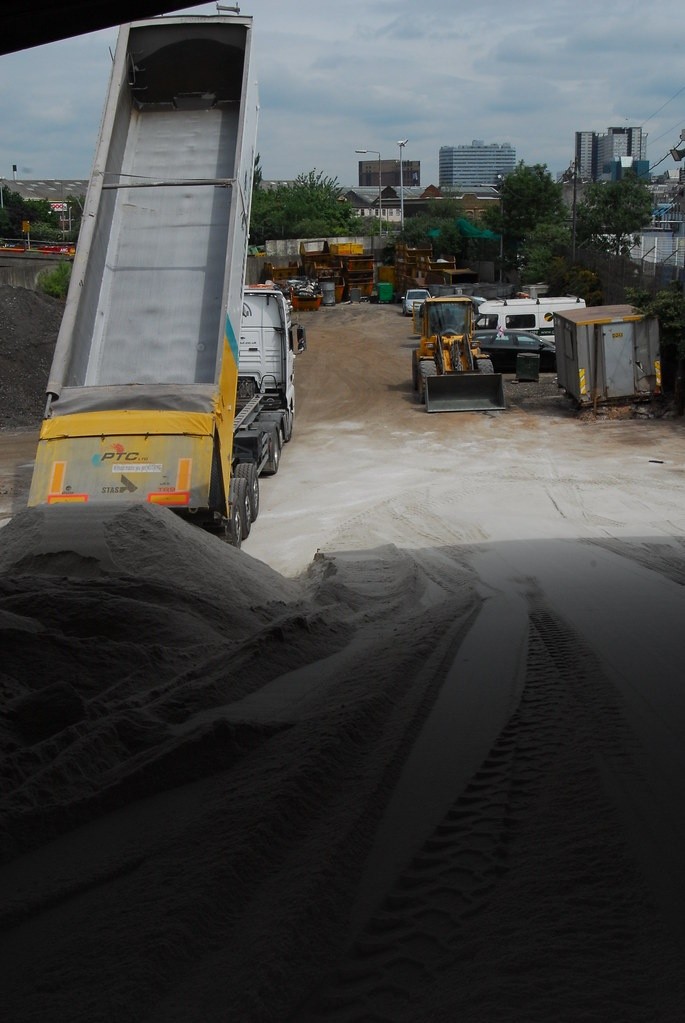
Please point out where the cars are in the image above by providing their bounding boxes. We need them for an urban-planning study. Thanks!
[471,329,557,372]
[401,289,432,316]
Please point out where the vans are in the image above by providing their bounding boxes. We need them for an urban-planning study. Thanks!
[472,297,586,343]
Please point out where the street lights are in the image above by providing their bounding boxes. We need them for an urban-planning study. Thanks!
[397,138,409,230]
[355,149,382,236]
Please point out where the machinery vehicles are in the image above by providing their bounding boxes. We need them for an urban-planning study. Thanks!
[412,295,507,412]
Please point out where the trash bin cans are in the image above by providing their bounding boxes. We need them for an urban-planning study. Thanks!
[376,281,393,304]
[320,282,336,306]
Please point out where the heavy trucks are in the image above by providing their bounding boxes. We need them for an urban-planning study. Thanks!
[27,1,306,547]
